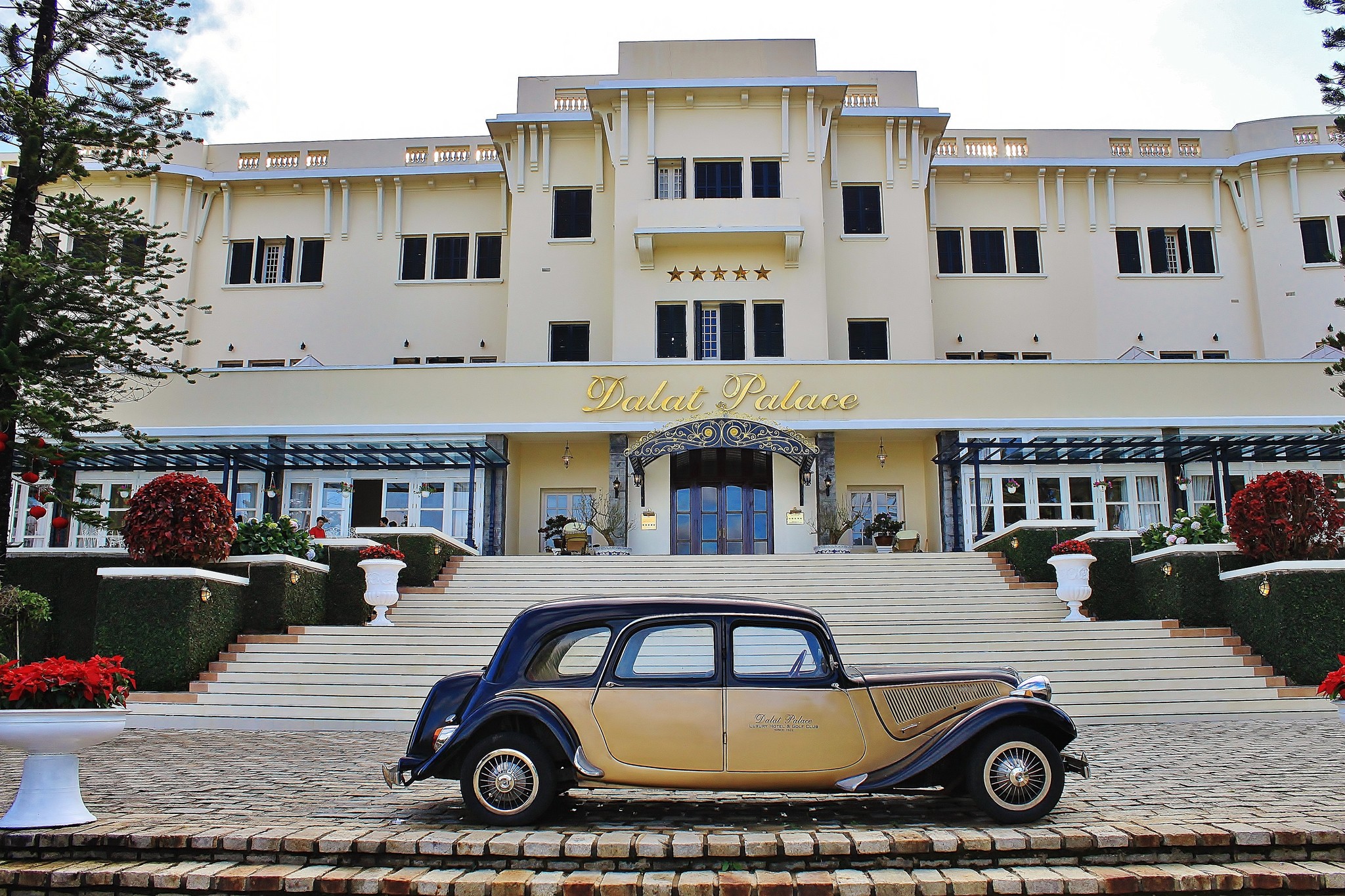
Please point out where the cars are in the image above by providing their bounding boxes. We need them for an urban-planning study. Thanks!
[381,594,1092,827]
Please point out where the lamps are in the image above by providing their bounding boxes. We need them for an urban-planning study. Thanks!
[955,332,1218,343]
[228,338,485,352]
[291,570,301,585]
[952,472,961,488]
[434,542,442,554]
[1175,476,1179,480]
[1161,562,1172,576]
[613,464,645,498]
[877,436,888,467]
[562,440,574,470]
[803,465,832,496]
[1259,579,1270,596]
[1010,537,1019,548]
[201,586,212,602]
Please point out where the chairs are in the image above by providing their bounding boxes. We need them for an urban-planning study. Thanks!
[561,522,589,555]
[892,530,920,553]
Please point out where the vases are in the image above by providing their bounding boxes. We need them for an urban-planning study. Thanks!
[1047,553,1097,622]
[357,559,407,625]
[1179,484,1188,491]
[1008,486,1016,493]
[421,491,430,498]
[0,709,134,831]
[1098,485,1106,492]
[120,491,129,498]
[267,491,276,498]
[874,536,894,546]
[342,491,351,498]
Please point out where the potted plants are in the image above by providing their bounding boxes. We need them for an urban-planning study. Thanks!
[804,494,876,553]
[567,488,638,555]
[538,514,577,549]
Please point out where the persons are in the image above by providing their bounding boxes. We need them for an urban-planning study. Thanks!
[309,517,326,539]
[389,521,397,527]
[379,517,391,538]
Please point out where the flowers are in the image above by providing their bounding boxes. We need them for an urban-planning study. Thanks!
[264,485,281,497]
[863,511,905,537]
[413,483,437,494]
[1176,478,1192,486]
[1006,479,1020,489]
[0,654,137,709]
[1051,539,1092,556]
[336,481,355,494]
[117,487,132,494]
[360,544,405,559]
[1093,480,1113,488]
